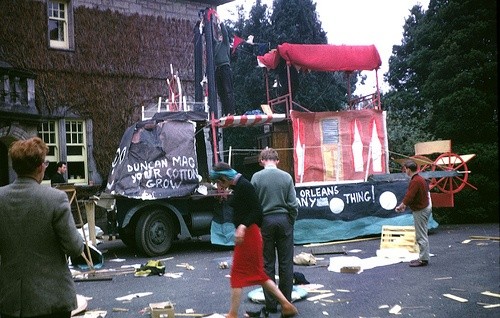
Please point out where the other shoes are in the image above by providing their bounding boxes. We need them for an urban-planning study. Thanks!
[279,304,302,318]
[261,303,278,315]
[218,311,243,318]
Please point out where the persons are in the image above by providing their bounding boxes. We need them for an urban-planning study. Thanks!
[250,149,299,313]
[0,137,84,318]
[211,15,238,116]
[51,162,67,183]
[395,161,432,267]
[209,162,299,318]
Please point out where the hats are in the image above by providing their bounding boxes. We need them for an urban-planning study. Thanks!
[207,162,238,179]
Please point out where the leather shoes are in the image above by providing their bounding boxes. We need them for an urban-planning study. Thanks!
[408,258,429,267]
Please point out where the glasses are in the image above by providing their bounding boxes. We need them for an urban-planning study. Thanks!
[37,159,51,170]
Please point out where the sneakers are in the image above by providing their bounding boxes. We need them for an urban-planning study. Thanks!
[227,110,238,118]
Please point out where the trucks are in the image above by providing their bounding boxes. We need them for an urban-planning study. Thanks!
[104,6,434,258]
